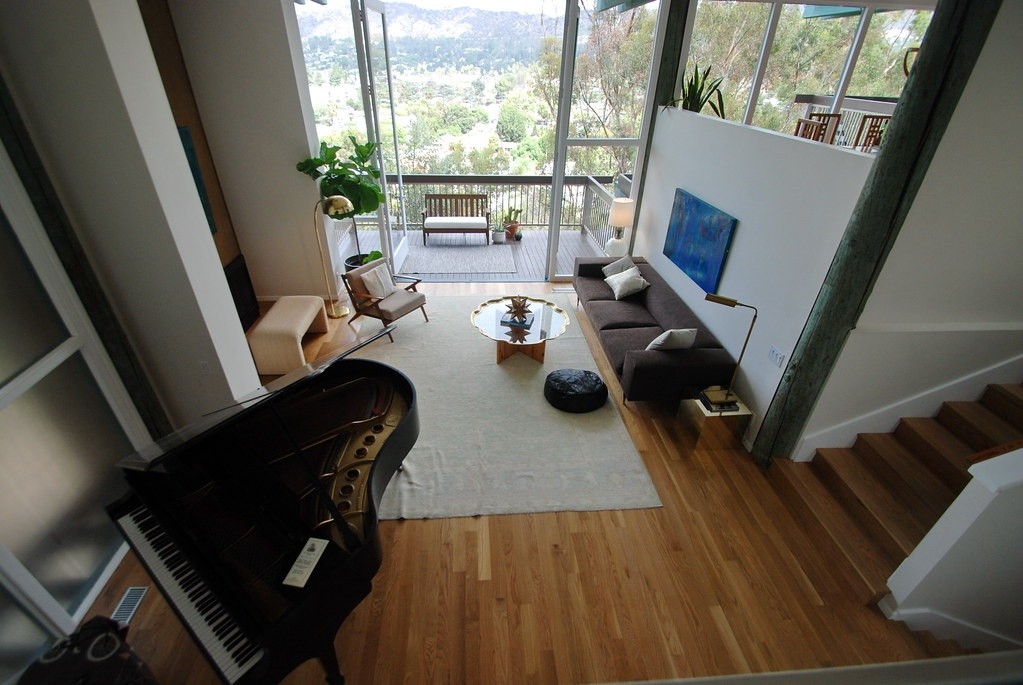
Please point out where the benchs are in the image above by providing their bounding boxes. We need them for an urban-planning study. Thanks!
[417,194,491,248]
[249,295,330,375]
[572,256,725,405]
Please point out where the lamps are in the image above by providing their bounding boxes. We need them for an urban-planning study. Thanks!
[313,195,355,319]
[698,292,758,412]
[604,198,635,256]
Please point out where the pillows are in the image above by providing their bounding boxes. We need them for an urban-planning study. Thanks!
[645,328,698,351]
[604,266,651,300]
[603,254,642,276]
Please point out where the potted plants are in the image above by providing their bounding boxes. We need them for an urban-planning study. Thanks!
[515,230,522,241]
[296,135,386,272]
[489,215,511,244]
[502,206,523,238]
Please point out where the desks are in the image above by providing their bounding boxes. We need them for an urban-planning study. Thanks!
[470,295,571,365]
[672,394,752,451]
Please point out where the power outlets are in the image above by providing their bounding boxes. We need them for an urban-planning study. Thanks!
[749,410,757,425]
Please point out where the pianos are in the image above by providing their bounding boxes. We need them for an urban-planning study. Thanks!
[101,321,422,685]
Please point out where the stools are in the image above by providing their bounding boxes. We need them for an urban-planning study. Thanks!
[17,615,159,685]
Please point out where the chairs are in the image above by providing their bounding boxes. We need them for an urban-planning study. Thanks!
[807,112,841,144]
[340,256,429,343]
[854,114,891,148]
[792,117,823,142]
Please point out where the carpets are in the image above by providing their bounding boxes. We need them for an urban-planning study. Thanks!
[346,294,667,524]
[400,245,518,274]
